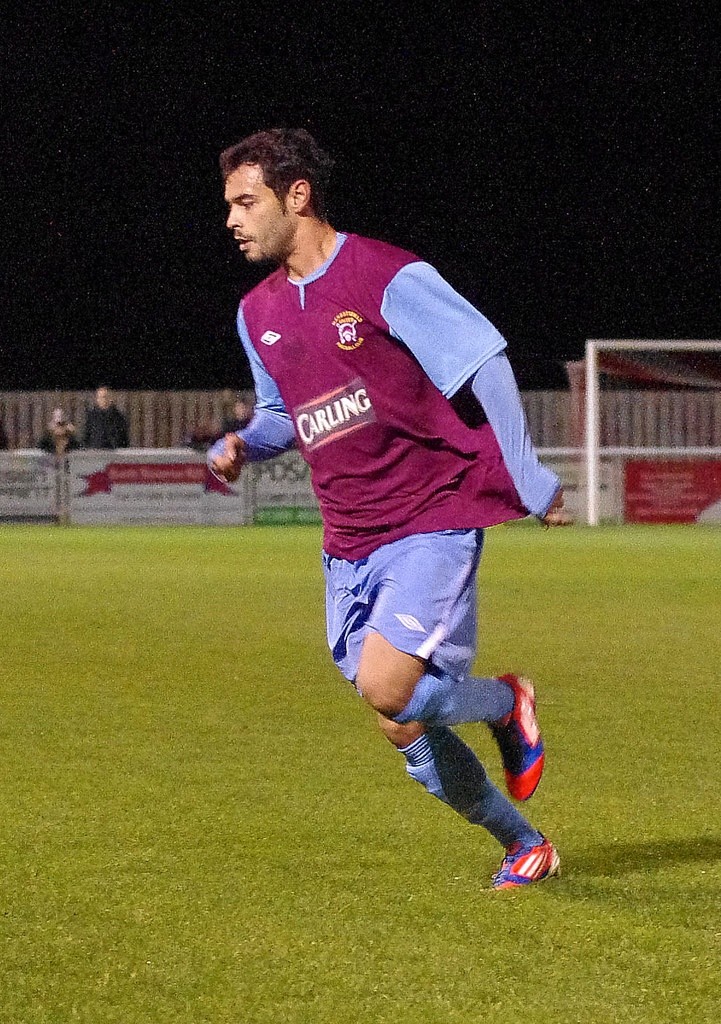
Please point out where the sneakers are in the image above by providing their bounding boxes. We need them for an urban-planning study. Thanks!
[490,831,562,891]
[487,673,545,801]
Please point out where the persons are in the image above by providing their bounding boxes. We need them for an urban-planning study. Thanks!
[37,408,81,452]
[83,387,129,449]
[206,129,575,890]
[224,398,250,432]
